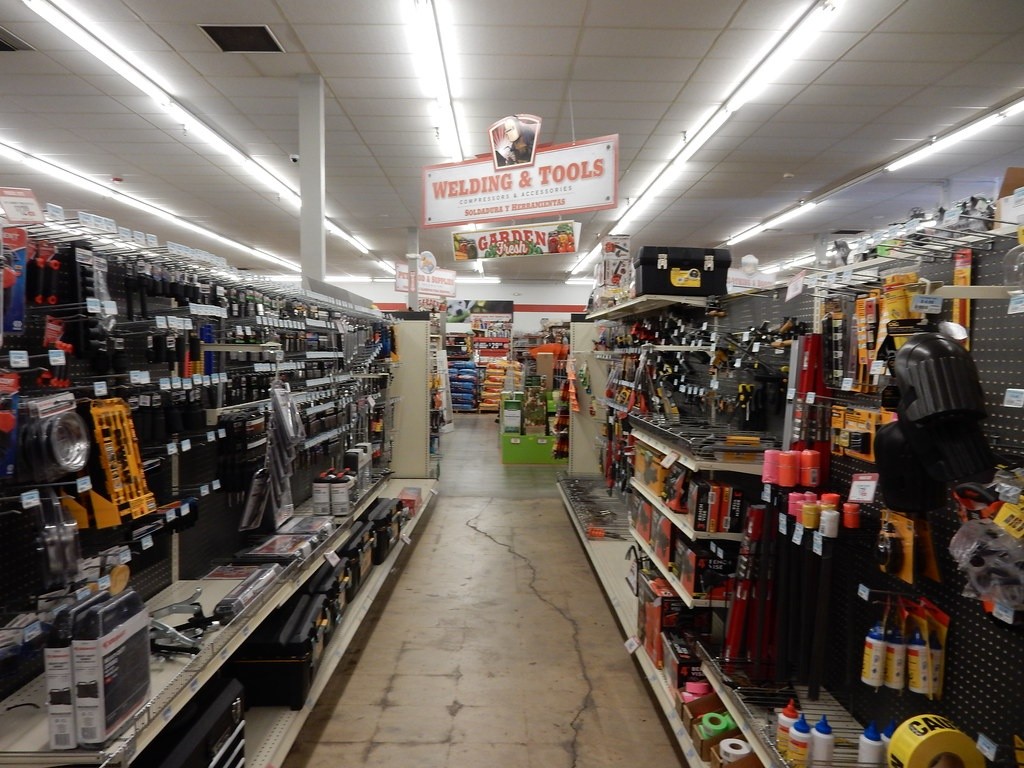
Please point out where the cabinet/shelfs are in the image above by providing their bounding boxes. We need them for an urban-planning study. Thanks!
[583,293,718,414]
[1,476,434,768]
[429,334,554,479]
[139,303,402,449]
[558,425,890,767]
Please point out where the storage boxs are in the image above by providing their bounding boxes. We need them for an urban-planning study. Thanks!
[127,487,419,768]
[627,441,756,667]
[633,244,733,297]
[497,374,567,438]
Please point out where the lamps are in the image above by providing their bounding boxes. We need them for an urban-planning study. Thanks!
[1,1,1024,279]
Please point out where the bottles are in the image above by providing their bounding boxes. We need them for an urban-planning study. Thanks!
[786,713,813,768]
[860,622,943,694]
[810,714,834,768]
[880,721,897,768]
[776,698,801,754]
[858,721,884,768]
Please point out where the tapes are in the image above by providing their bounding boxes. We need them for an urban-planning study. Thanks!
[698,712,737,740]
[681,682,716,703]
[720,738,752,763]
[886,714,985,768]
